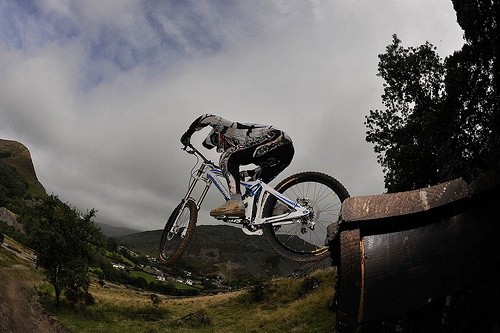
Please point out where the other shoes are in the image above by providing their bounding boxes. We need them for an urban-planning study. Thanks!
[210,197,246,216]
[272,204,289,215]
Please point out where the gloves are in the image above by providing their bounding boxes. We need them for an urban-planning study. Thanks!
[180,132,191,146]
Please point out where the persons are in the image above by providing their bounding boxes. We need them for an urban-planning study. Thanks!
[181,114,295,232]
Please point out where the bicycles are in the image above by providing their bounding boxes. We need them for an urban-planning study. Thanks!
[157,142,351,268]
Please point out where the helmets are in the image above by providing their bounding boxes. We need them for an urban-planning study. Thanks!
[210,129,219,146]
